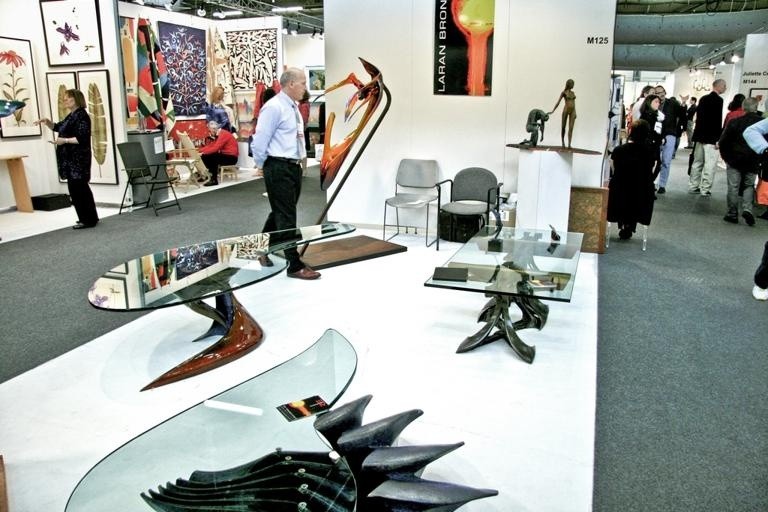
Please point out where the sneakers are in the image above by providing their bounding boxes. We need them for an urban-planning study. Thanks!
[702,191,711,196]
[751,285,768,301]
[689,188,700,194]
[724,216,738,224]
[742,211,755,225]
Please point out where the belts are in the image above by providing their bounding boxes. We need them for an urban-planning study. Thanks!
[276,158,302,165]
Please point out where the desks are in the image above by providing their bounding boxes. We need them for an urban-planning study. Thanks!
[87,222,357,392]
[0,154,34,212]
[424,221,584,364]
[66,324,499,512]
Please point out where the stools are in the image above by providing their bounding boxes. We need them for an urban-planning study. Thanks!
[218,165,238,182]
[163,147,200,188]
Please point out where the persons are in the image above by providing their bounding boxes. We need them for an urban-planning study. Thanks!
[32,89,100,230]
[261,89,276,199]
[204,87,236,133]
[196,121,239,187]
[297,88,311,176]
[250,66,321,280]
[547,79,577,149]
[605,80,767,302]
[519,109,549,147]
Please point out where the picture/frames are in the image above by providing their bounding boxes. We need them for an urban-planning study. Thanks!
[0,35,42,138]
[44,71,77,184]
[564,186,607,254]
[74,69,119,185]
[38,0,104,67]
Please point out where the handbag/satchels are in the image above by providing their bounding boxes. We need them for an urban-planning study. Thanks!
[756,178,768,206]
[688,154,693,176]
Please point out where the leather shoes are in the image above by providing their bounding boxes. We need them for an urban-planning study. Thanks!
[287,266,321,280]
[204,179,218,186]
[657,187,666,193]
[258,255,273,266]
[73,222,89,229]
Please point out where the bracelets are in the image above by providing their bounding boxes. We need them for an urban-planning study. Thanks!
[64,137,69,144]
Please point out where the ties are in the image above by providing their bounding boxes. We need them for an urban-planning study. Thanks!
[293,102,304,160]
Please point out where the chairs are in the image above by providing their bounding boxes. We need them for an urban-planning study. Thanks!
[115,141,180,217]
[379,157,438,247]
[434,164,503,250]
